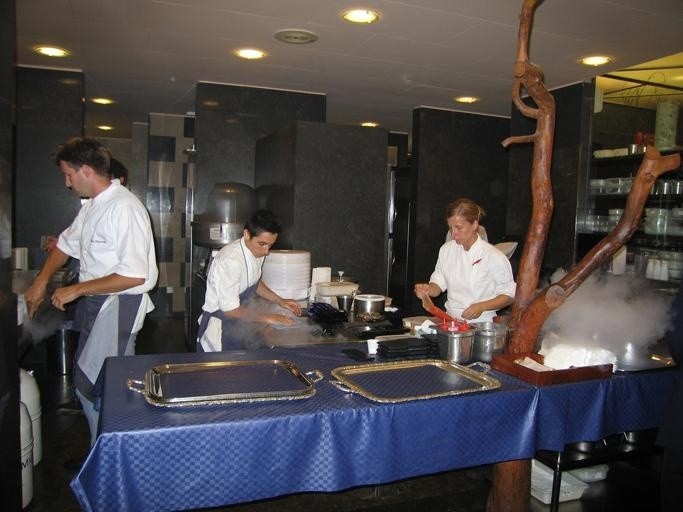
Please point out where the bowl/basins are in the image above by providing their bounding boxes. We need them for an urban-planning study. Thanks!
[353,294,385,318]
[667,250,683,279]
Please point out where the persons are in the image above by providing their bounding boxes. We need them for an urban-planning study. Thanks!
[446,225,488,243]
[23,136,159,449]
[197,208,302,353]
[415,198,517,324]
[43,159,157,355]
[389,198,408,266]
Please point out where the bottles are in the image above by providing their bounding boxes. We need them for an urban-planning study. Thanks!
[441,317,470,332]
[644,258,669,282]
[635,129,644,147]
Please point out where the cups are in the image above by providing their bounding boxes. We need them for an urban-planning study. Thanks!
[629,143,637,155]
[336,294,353,312]
[658,178,683,195]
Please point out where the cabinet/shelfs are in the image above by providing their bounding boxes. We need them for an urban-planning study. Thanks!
[573,145,683,287]
